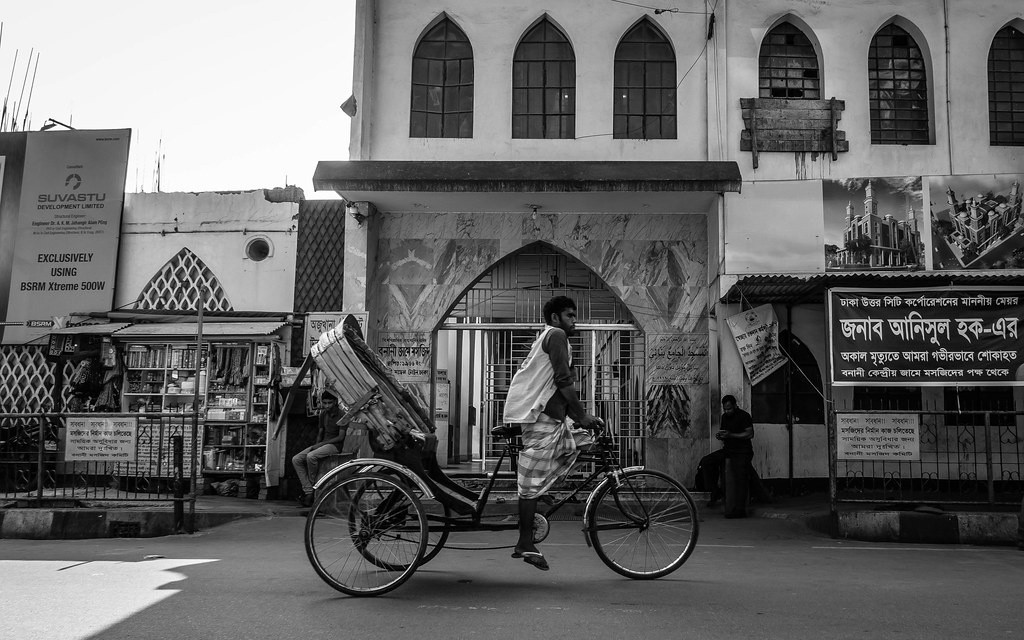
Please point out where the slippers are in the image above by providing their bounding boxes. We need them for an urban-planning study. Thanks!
[537,497,561,505]
[512,551,549,571]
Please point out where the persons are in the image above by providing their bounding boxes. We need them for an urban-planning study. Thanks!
[707,394,756,509]
[501,296,606,571]
[291,389,349,511]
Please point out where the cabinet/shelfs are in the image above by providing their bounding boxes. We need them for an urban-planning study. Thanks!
[121,342,285,476]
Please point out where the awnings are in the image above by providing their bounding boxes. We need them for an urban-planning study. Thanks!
[111,320,291,338]
[46,319,130,335]
[719,273,1024,304]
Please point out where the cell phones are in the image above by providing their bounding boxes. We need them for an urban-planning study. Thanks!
[718,430,726,436]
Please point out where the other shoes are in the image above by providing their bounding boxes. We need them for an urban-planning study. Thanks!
[707,493,721,507]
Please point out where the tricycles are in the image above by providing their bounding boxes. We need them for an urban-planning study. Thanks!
[272,311,701,598]
